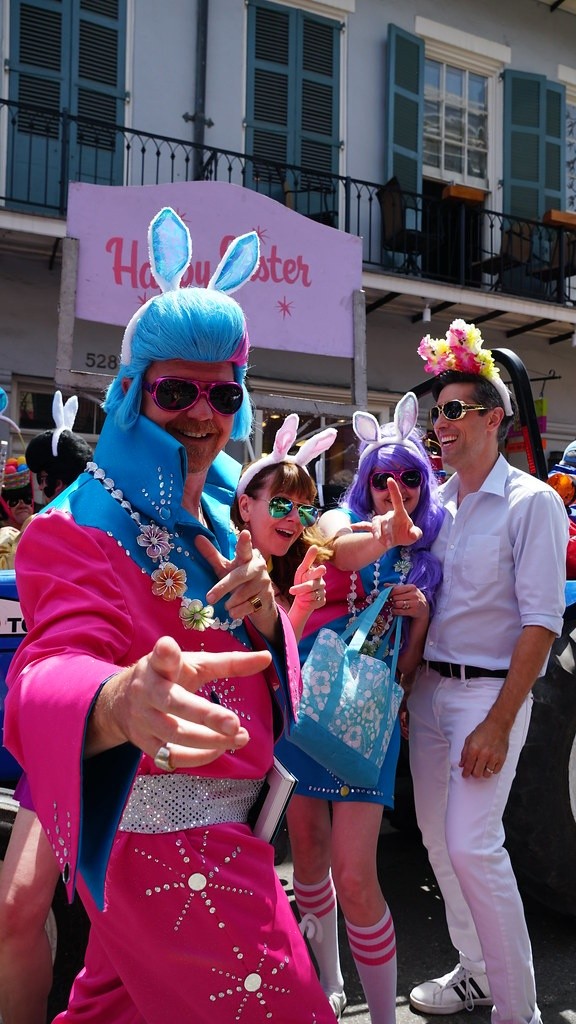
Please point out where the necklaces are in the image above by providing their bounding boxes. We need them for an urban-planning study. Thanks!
[84,461,245,631]
[349,548,412,657]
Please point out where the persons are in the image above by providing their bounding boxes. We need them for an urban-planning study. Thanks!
[231,414,337,647]
[2,205,337,1024]
[399,319,570,1024]
[287,393,442,1024]
[0,393,94,1024]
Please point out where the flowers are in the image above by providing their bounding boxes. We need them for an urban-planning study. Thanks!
[416,318,501,381]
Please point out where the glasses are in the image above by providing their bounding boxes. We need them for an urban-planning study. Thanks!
[430,399,493,426]
[369,469,423,491]
[7,499,31,511]
[254,496,319,527]
[142,376,243,417]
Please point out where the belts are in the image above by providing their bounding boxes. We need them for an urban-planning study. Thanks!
[424,659,509,679]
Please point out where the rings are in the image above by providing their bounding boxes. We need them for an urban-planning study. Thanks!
[403,601,409,609]
[249,597,262,612]
[155,747,175,772]
[486,768,494,774]
[389,607,393,614]
[315,591,321,601]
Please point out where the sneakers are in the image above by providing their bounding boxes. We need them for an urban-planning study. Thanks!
[409,964,494,1015]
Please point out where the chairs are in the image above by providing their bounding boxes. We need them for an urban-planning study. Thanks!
[282,181,339,228]
[478,216,540,295]
[374,175,442,277]
[532,234,576,307]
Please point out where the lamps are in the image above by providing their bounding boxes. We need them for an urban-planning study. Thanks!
[422,303,431,323]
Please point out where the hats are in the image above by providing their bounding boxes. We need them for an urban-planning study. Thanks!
[106,206,261,442]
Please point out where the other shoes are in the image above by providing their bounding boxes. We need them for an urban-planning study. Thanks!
[322,990,347,1021]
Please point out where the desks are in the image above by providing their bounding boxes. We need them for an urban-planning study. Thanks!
[441,184,484,288]
[543,209,576,306]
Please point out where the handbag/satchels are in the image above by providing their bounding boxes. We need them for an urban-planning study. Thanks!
[284,583,404,789]
